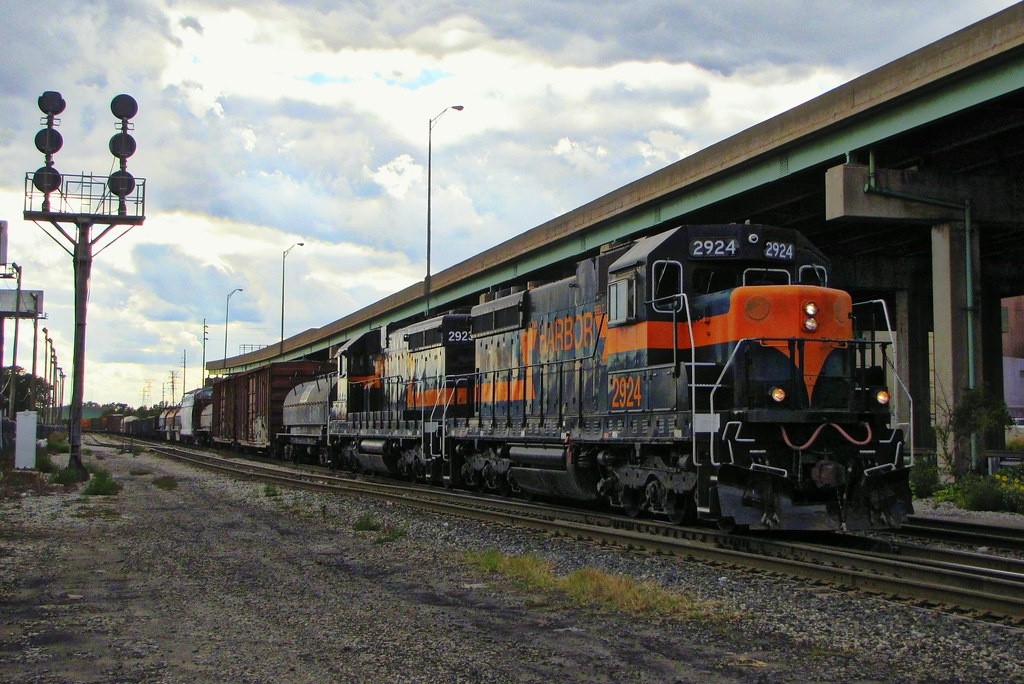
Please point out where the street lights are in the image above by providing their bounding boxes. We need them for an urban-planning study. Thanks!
[424,105,464,292]
[224,289,243,367]
[281,242,303,351]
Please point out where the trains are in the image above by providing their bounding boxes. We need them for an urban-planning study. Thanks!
[64,219,916,538]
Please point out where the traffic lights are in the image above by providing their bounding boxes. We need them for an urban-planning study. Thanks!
[38,91,66,115]
[108,171,135,196]
[34,167,61,193]
[111,94,138,120]
[34,129,63,153]
[109,133,137,158]
